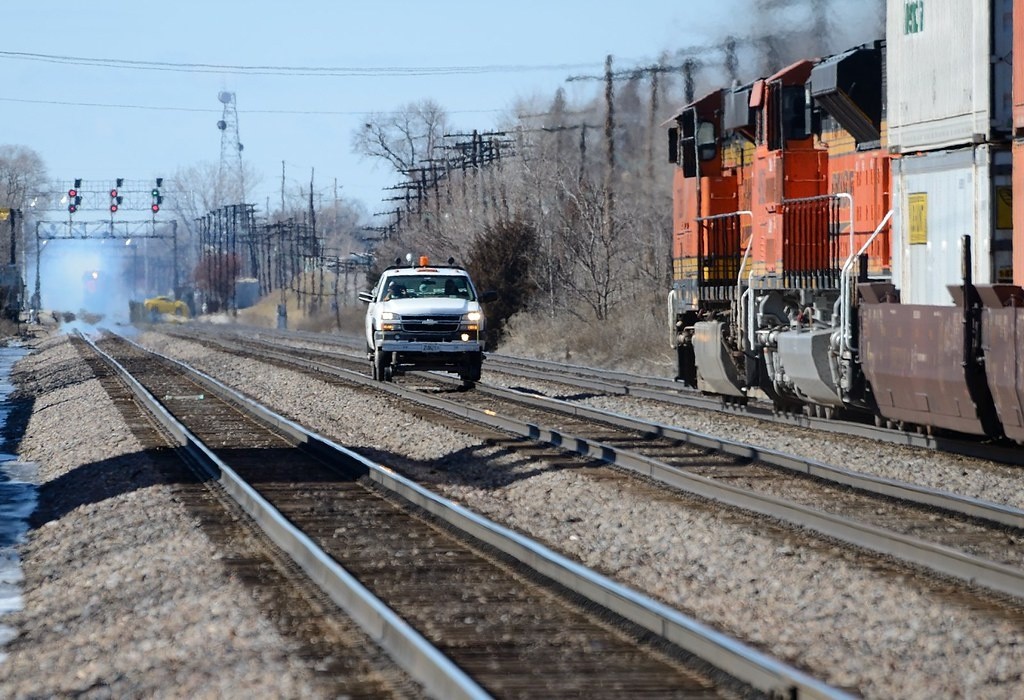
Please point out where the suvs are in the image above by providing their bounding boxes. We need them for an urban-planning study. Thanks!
[355,255,486,385]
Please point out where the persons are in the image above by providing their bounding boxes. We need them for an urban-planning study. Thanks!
[383,281,409,301]
[444,280,465,299]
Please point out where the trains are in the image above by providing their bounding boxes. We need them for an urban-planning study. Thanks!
[662,4,1024,450]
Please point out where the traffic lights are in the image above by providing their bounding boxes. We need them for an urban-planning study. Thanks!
[68,204,76,213]
[152,189,158,196]
[111,205,117,212]
[152,204,159,212]
[68,190,76,197]
[110,189,117,197]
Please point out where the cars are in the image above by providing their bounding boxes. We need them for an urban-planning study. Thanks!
[143,296,189,317]
